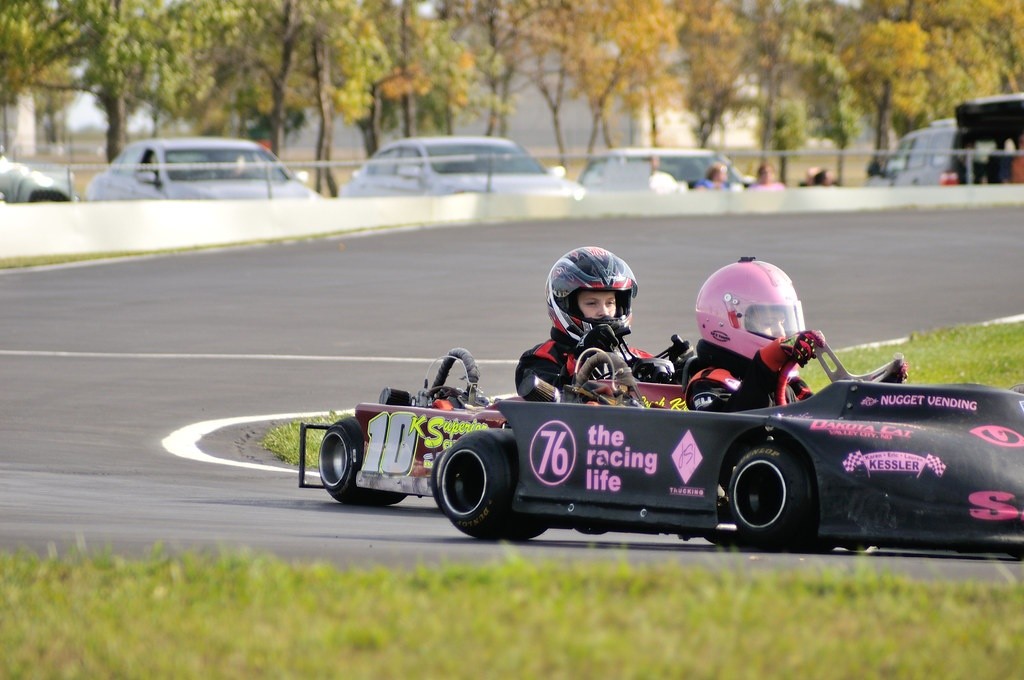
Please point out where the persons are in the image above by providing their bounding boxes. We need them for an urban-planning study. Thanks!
[813,170,839,187]
[798,166,823,187]
[515,246,672,404]
[864,159,891,185]
[683,255,908,419]
[694,164,731,191]
[748,162,786,191]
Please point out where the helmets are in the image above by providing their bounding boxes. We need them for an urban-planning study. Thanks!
[695,255,808,378]
[545,246,640,338]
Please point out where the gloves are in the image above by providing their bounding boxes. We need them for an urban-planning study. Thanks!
[669,340,694,376]
[881,361,909,383]
[759,331,826,371]
[573,322,619,360]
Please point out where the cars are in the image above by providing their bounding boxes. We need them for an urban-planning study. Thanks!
[86,135,325,199]
[0,149,75,203]
[571,148,753,189]
[339,135,583,196]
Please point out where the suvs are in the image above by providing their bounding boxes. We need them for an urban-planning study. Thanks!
[863,92,1024,186]
